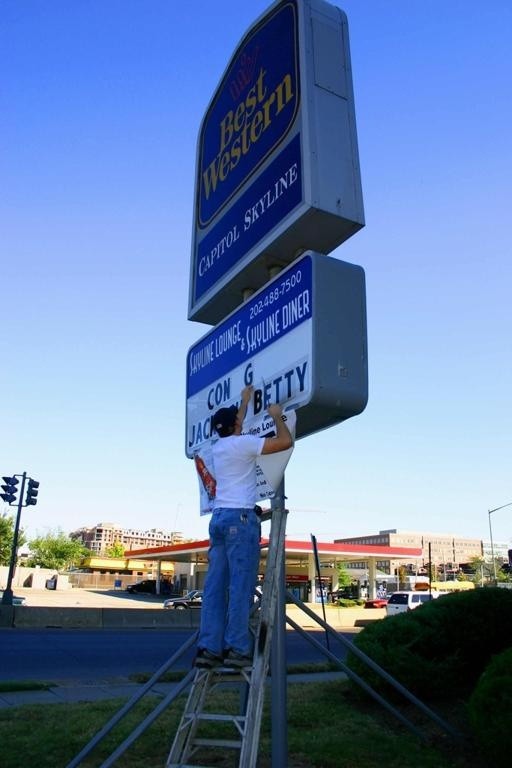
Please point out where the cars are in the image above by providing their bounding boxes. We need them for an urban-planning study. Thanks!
[386,590,451,617]
[126,580,174,594]
[164,589,203,610]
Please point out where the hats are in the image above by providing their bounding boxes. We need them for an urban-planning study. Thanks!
[213,405,238,432]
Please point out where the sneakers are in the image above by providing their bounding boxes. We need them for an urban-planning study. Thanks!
[193,648,253,668]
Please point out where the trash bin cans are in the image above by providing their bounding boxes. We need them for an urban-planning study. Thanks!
[47,578,56,589]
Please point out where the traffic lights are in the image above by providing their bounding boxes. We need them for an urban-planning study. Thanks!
[0,477,19,503]
[25,478,40,505]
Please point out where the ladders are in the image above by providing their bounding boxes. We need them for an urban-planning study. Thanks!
[166,508,290,767]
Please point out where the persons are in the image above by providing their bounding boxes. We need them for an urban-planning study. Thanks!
[193,385,294,668]
